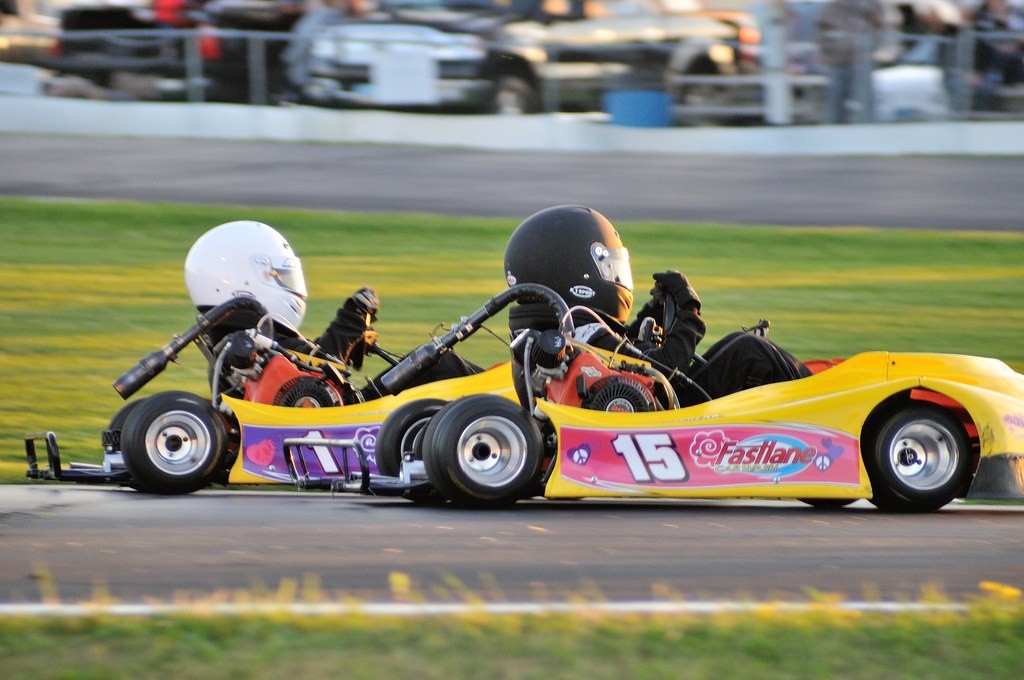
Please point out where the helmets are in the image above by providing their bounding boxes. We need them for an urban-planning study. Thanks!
[181,217,310,341]
[503,202,635,330]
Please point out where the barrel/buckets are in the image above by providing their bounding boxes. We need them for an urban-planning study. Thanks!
[608,92,672,127]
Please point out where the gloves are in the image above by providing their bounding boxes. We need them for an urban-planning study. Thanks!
[647,269,701,316]
[347,286,380,324]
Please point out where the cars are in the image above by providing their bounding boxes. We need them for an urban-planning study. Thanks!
[0,0,1023,119]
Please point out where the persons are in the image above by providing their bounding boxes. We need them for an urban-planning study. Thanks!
[185,221,487,408]
[504,204,813,413]
